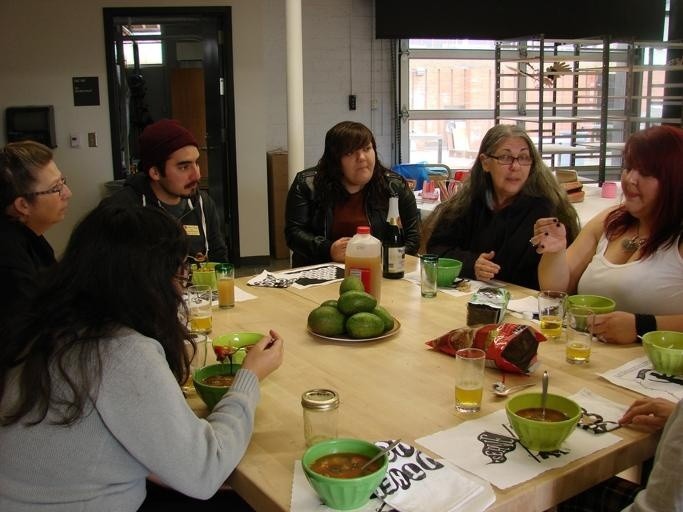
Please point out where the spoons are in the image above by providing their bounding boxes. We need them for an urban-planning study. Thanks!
[490,380,535,398]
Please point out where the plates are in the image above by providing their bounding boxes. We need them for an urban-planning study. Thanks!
[306,316,402,346]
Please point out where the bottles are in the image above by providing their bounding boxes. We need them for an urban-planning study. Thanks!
[383,193,406,280]
[300,388,339,446]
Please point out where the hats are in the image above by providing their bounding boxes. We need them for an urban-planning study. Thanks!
[132,118,199,161]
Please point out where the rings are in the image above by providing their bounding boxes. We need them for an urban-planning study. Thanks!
[531,243,537,247]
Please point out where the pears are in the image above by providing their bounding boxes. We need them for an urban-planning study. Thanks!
[308,277,396,338]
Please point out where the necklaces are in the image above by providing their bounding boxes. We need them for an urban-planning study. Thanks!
[623,225,652,250]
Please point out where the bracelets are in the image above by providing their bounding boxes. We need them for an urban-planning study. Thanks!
[633,310,659,341]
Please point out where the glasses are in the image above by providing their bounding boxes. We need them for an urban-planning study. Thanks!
[481,153,535,166]
[170,270,193,288]
[27,175,66,197]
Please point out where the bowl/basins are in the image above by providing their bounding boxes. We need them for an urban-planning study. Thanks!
[302,438,390,511]
[640,329,683,378]
[193,362,243,412]
[431,258,462,289]
[564,293,615,333]
[185,262,223,288]
[213,333,267,366]
[504,392,582,454]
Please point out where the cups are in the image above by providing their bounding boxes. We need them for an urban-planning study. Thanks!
[215,262,236,310]
[455,348,483,414]
[187,284,213,333]
[179,333,207,398]
[563,306,592,365]
[418,253,438,298]
[538,290,565,339]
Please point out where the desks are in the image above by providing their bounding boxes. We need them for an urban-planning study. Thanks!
[386,180,627,230]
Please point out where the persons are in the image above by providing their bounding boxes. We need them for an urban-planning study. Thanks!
[529,123,682,345]
[616,395,682,512]
[422,124,583,296]
[0,141,74,346]
[280,120,419,267]
[0,193,286,511]
[64,117,229,268]
